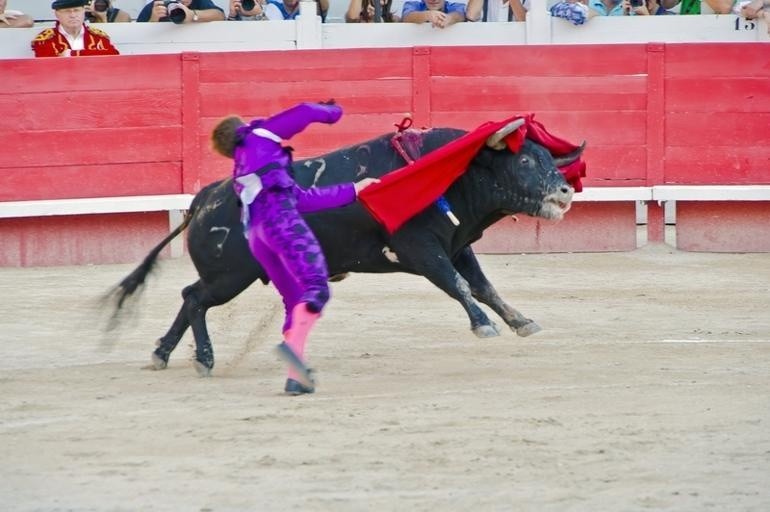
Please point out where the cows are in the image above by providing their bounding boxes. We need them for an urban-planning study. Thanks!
[95,114,588,380]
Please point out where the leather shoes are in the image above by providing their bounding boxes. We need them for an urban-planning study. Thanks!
[272,342,316,396]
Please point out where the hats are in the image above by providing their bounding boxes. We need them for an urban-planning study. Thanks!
[50,0,89,9]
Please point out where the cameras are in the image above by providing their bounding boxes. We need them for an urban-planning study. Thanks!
[93,0,108,11]
[236,0,255,10]
[627,0,648,12]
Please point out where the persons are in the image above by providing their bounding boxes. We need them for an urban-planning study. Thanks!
[31,0,120,55]
[0,0,33,28]
[211,98,381,394]
